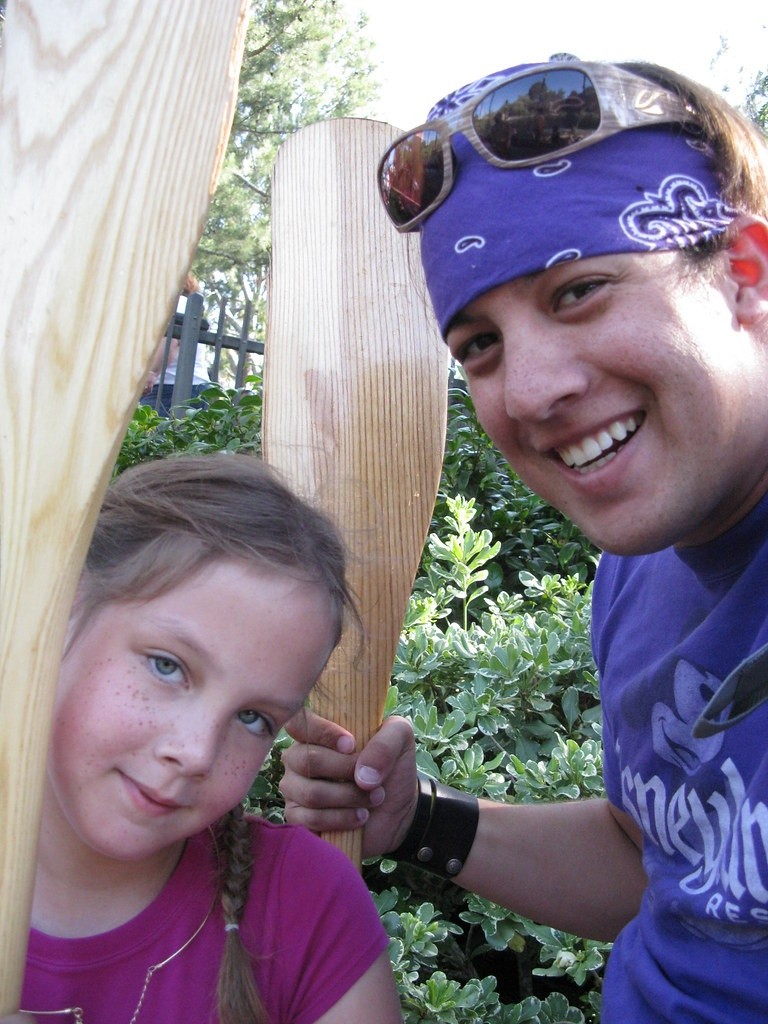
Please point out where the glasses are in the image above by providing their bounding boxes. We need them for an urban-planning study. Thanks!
[376,62,704,234]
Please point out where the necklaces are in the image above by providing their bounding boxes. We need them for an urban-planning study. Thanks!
[16,825,221,1024]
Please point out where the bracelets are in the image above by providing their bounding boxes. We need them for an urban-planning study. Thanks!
[384,768,480,882]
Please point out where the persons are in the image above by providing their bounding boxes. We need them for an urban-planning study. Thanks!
[282,52,768,1024]
[490,111,512,157]
[137,271,219,417]
[550,125,560,144]
[0,452,402,1024]
[564,90,583,133]
[238,390,255,401]
[534,108,545,141]
